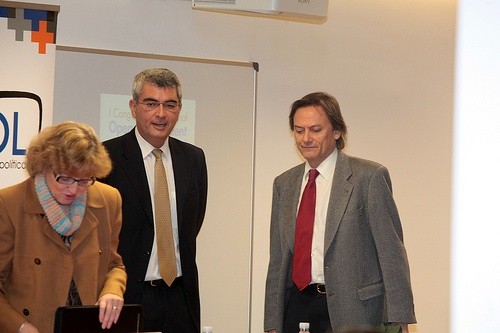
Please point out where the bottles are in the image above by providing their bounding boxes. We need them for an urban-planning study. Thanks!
[298,322,311,333]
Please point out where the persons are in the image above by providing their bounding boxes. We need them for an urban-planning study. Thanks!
[263,92,418,333]
[91,67,210,333]
[0,122,128,333]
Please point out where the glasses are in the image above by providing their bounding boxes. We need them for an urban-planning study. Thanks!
[136,99,181,114]
[52,168,96,188]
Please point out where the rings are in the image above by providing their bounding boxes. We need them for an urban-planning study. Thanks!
[112,306,118,310]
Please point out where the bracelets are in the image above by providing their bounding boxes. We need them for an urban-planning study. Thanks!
[20,322,26,329]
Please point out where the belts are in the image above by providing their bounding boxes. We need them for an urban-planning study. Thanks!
[303,284,326,297]
[145,279,165,288]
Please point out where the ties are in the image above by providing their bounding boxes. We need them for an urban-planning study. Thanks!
[152,149,178,287]
[292,170,319,291]
